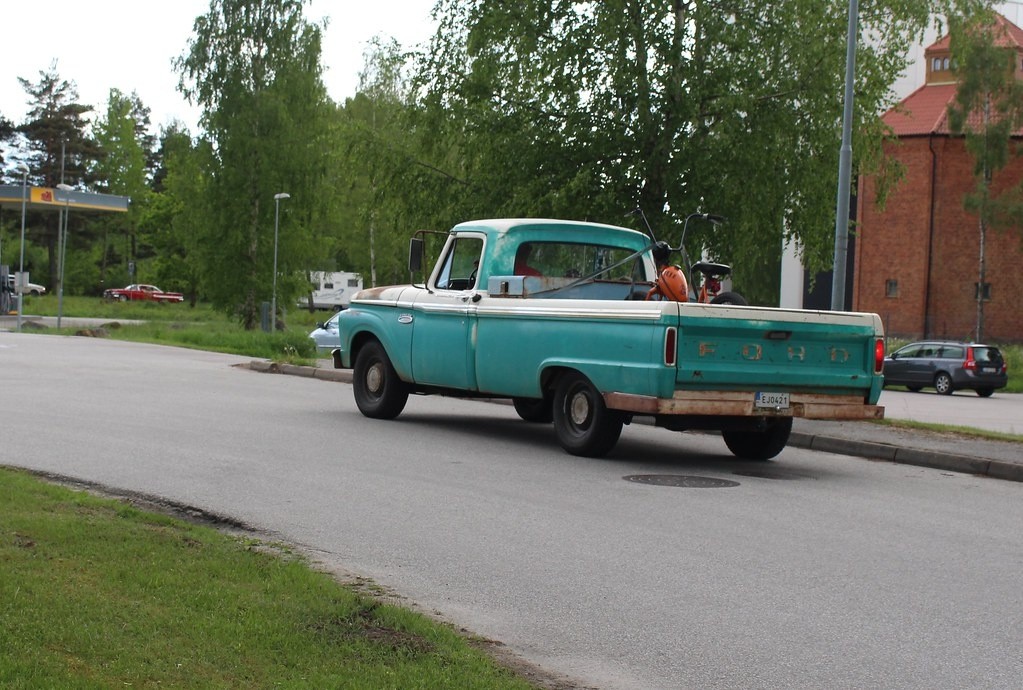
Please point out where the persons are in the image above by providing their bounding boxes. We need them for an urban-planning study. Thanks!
[514,243,541,276]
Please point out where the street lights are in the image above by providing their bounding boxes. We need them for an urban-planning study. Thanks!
[56,183,75,330]
[14,164,29,333]
[271,192,290,334]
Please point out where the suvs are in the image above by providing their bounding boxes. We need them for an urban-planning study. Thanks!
[882,338,1008,398]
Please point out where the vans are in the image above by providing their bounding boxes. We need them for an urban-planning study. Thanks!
[294,271,363,312]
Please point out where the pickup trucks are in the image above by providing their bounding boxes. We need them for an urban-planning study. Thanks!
[330,217,887,461]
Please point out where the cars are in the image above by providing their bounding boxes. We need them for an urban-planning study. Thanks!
[309,308,343,368]
[103,283,184,306]
[8,274,46,298]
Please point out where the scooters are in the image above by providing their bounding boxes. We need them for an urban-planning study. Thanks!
[623,206,753,309]
